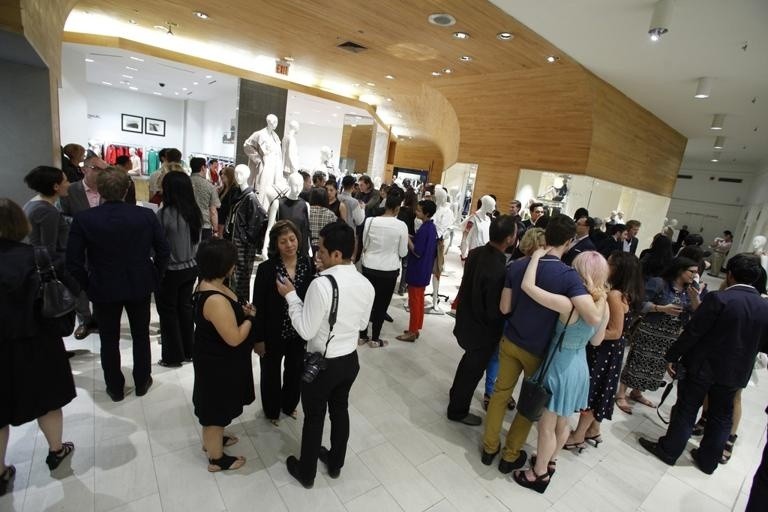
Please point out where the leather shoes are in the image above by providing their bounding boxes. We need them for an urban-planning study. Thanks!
[104,384,125,403]
[319,443,341,479]
[357,335,373,345]
[498,449,528,474]
[690,447,715,474]
[638,436,676,466]
[481,437,501,466]
[73,317,92,339]
[447,411,482,426]
[286,455,315,489]
[395,332,416,343]
[404,329,420,339]
[135,376,153,397]
[369,339,389,348]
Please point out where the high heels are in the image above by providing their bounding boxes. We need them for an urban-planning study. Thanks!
[531,453,557,478]
[396,286,409,296]
[690,410,708,436]
[290,408,298,421]
[585,433,604,448]
[562,441,587,453]
[270,416,280,427]
[513,467,551,494]
[717,433,738,465]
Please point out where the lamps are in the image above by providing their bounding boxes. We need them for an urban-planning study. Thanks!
[709,113,725,130]
[648,27,668,41]
[710,152,721,162]
[712,136,726,150]
[694,78,712,99]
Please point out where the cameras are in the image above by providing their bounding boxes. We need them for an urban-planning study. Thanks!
[671,361,688,379]
[300,352,330,384]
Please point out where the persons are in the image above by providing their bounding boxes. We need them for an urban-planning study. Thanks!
[281,120,300,182]
[243,113,290,214]
[1,144,768,498]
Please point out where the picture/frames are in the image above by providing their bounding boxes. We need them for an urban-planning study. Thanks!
[120,112,144,134]
[144,116,166,137]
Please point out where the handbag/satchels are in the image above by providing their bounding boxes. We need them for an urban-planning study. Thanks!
[34,278,80,320]
[432,234,445,275]
[516,376,553,425]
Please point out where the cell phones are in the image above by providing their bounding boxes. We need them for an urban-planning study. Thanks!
[277,272,284,284]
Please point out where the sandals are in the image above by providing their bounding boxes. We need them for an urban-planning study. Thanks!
[1,463,16,495]
[614,394,633,415]
[508,395,516,410]
[45,440,74,470]
[206,452,247,473]
[483,392,491,411]
[630,390,658,408]
[202,434,239,451]
